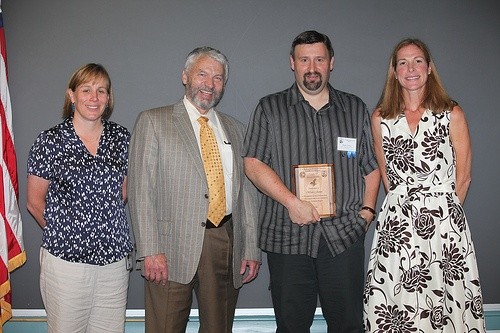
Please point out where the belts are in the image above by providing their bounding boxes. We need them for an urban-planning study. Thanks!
[206,213,233,228]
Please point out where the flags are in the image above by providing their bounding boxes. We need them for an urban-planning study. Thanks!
[0,0,27,333]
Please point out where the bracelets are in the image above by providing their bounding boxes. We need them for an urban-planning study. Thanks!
[362,206,376,221]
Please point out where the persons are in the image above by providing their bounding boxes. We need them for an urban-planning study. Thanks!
[26,62,131,333]
[127,47,263,333]
[241,30,381,333]
[362,39,487,333]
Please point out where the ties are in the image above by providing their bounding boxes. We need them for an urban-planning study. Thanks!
[197,116,226,228]
